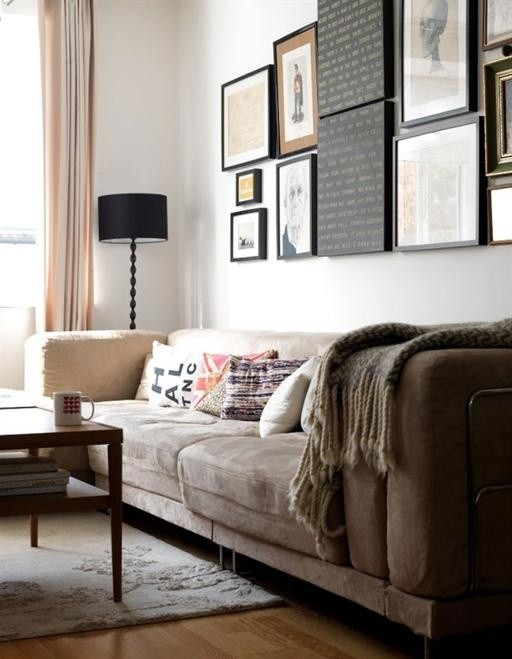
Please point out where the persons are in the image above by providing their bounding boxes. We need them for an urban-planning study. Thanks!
[291,63,304,123]
[282,165,306,255]
[413,0,448,97]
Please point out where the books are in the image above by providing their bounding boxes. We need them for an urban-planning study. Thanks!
[0,469,70,481]
[0,476,68,490]
[0,456,59,475]
[0,485,67,497]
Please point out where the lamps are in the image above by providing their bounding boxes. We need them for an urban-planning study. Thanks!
[98,192,168,329]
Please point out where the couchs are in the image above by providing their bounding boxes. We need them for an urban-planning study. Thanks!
[22,328,512,659]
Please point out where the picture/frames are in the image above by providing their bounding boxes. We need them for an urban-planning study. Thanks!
[276,153,317,261]
[482,57,512,177]
[391,115,488,253]
[221,65,277,173]
[486,182,512,246]
[230,208,267,262]
[398,0,478,129]
[272,18,318,160]
[480,0,512,51]
[236,168,262,206]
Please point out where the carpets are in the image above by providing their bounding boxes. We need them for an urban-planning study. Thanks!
[0,508,286,643]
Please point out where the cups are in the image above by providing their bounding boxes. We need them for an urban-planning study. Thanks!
[53,391,96,426]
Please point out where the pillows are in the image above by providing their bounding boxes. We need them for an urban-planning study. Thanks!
[300,364,316,438]
[150,339,198,410]
[193,350,280,411]
[259,355,322,443]
[195,370,224,418]
[135,352,152,401]
[222,355,307,421]
